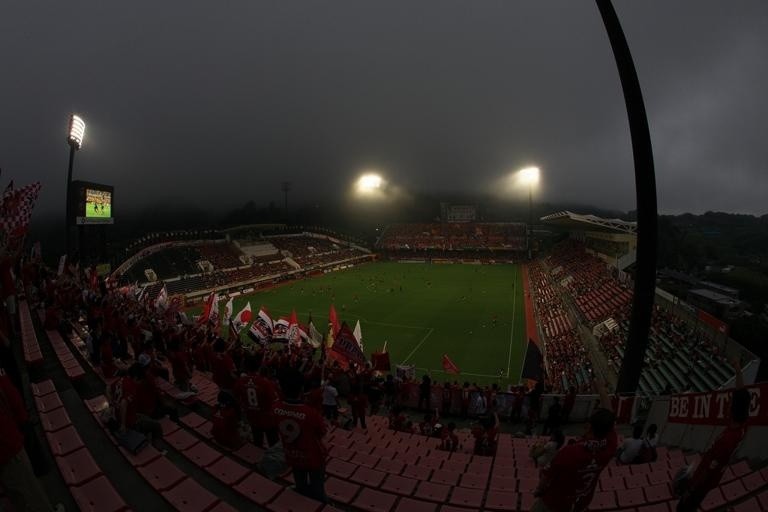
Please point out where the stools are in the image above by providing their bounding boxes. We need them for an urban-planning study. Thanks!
[20,293,762,510]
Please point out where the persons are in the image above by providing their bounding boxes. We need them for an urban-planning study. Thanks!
[117,236,370,299]
[374,223,506,250]
[527,240,737,394]
[1,262,660,512]
